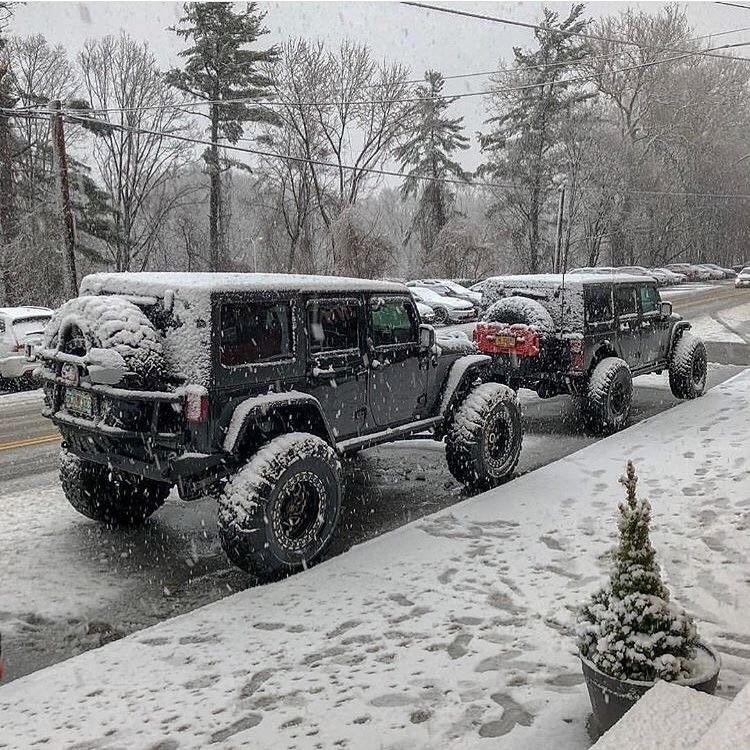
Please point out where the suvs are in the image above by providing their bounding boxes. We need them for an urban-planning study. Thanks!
[36,265,529,582]
[565,263,750,290]
[0,307,54,386]
[470,270,709,434]
[404,278,483,325]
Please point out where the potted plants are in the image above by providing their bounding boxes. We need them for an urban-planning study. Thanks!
[575,463,725,729]
